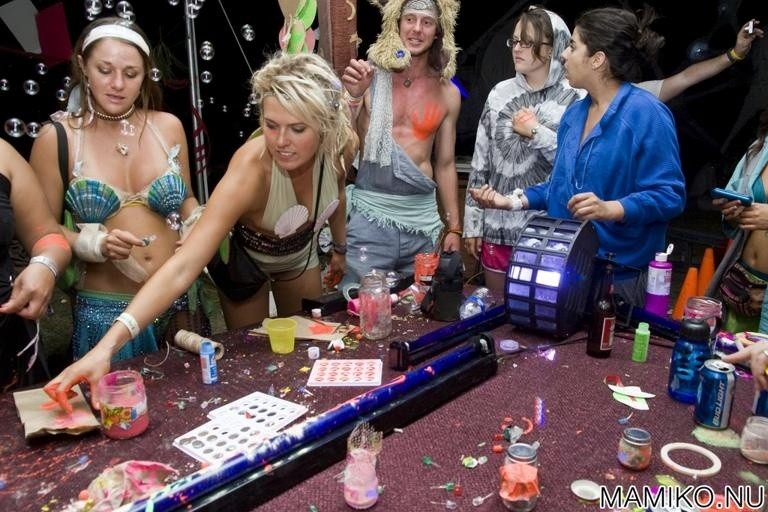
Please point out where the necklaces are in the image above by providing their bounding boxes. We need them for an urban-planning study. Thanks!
[400,71,435,88]
[87,107,136,121]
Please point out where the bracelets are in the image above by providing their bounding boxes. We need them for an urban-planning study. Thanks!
[445,229,463,236]
[504,189,528,211]
[29,256,61,279]
[730,49,746,62]
[344,90,362,107]
[114,311,141,341]
[333,242,348,255]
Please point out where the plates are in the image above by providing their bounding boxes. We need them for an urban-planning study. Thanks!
[570,480,600,501]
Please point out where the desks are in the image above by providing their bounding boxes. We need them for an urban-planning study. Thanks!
[0,272,768,511]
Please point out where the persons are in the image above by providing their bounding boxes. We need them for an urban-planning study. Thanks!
[712,109,768,335]
[463,5,588,299]
[28,21,205,371]
[46,55,357,413]
[471,9,683,309]
[722,336,768,391]
[343,1,462,304]
[1,138,69,388]
[632,18,764,105]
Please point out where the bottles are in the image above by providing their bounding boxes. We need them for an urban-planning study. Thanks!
[645,243,674,316]
[682,296,722,349]
[668,319,711,404]
[694,360,736,431]
[200,341,218,384]
[672,268,697,320]
[344,450,378,509]
[413,252,440,290]
[459,287,494,320]
[586,265,614,357]
[498,445,539,512]
[698,248,715,294]
[631,323,650,363]
[620,427,652,472]
[585,253,618,312]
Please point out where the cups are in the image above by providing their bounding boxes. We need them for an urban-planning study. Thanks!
[307,347,319,360]
[683,296,723,334]
[262,318,296,354]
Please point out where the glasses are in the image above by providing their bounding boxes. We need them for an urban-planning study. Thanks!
[507,38,553,48]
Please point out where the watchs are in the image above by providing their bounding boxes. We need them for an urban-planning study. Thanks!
[529,122,542,141]
[727,47,745,65]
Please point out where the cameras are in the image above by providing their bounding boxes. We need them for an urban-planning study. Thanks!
[743,20,757,35]
